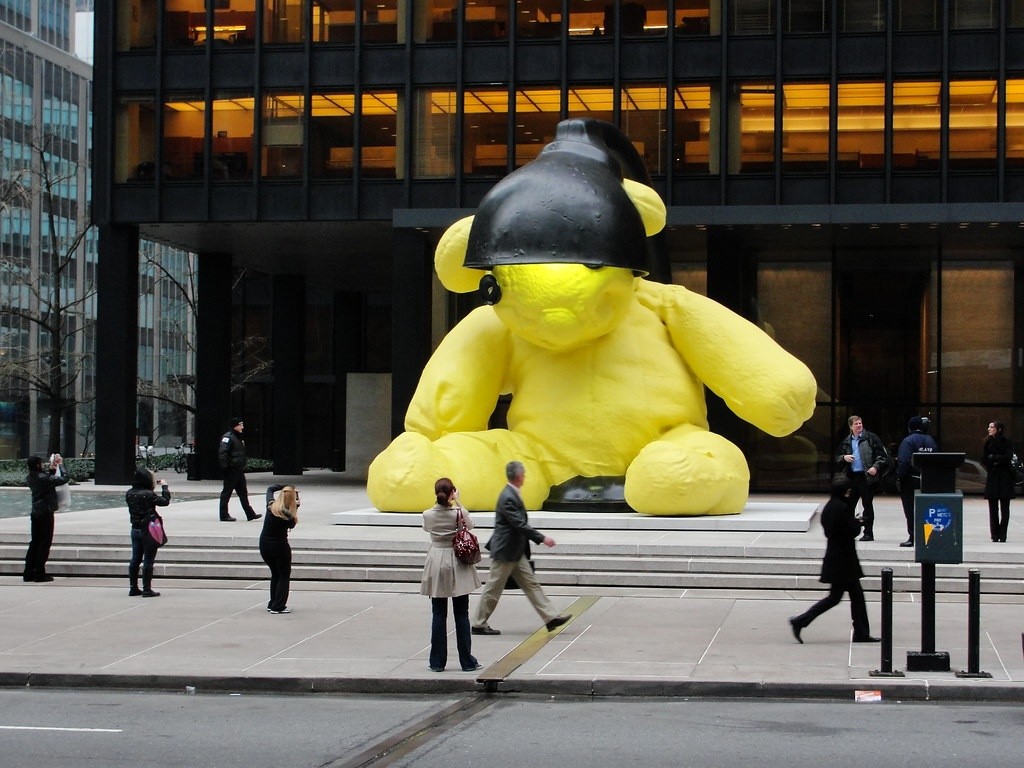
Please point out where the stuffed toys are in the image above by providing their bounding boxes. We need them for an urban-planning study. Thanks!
[366,115,818,516]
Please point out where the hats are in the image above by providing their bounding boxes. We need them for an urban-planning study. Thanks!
[908,416,922,431]
[231,418,243,429]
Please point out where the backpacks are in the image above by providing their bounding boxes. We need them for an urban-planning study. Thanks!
[867,431,894,481]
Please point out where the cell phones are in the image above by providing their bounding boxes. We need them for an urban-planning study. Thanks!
[156,480,161,485]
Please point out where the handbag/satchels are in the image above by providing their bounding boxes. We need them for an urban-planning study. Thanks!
[49,453,71,510]
[451,508,481,565]
[143,511,168,549]
[504,560,534,589]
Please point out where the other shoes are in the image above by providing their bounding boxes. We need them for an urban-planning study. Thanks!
[23,576,36,582]
[899,540,913,547]
[993,538,998,542]
[35,574,54,582]
[220,515,236,521]
[852,635,881,643]
[1000,537,1006,542]
[129,589,143,596]
[428,664,444,672]
[859,534,874,541]
[462,661,483,671]
[247,513,262,521]
[790,617,805,644]
[142,589,160,597]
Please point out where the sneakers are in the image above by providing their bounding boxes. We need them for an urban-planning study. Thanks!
[267,607,291,614]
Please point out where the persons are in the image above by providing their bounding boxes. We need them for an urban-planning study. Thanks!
[983,420,1014,543]
[126,465,171,598]
[217,418,263,522]
[836,415,889,541]
[422,479,483,672]
[790,472,881,643]
[896,416,938,547]
[22,454,71,582]
[258,482,301,613]
[470,461,572,635]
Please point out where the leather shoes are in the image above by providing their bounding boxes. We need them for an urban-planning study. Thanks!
[546,614,572,632]
[472,626,500,635]
